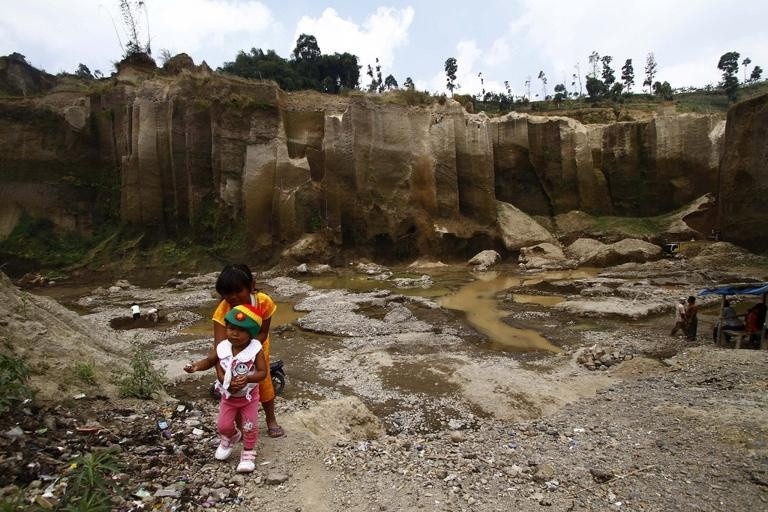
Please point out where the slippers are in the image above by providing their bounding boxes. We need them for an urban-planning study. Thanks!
[269,426,283,437]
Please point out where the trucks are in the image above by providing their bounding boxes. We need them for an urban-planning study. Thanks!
[660,240,691,259]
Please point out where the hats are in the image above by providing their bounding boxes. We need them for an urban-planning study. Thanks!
[225,303,263,339]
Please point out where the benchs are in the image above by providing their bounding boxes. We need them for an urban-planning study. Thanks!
[720,329,766,349]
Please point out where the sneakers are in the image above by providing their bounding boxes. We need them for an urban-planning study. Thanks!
[215,429,242,460]
[237,449,255,472]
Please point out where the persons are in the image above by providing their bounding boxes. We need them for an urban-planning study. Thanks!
[182,303,268,473]
[669,295,768,350]
[145,308,160,323]
[209,262,285,439]
[129,303,141,320]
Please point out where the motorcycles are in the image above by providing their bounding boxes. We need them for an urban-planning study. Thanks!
[208,359,288,403]
